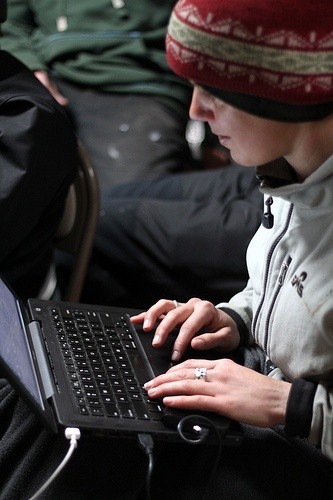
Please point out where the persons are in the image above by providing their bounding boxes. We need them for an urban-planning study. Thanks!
[0,0,333,500]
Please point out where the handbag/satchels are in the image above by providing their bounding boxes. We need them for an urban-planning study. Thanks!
[0,48,78,302]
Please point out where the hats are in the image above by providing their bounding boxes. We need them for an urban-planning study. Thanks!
[166,0,333,123]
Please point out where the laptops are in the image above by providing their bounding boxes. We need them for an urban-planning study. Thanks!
[0,275,247,448]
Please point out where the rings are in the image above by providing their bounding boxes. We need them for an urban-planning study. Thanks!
[194,367,207,381]
[173,299,179,309]
